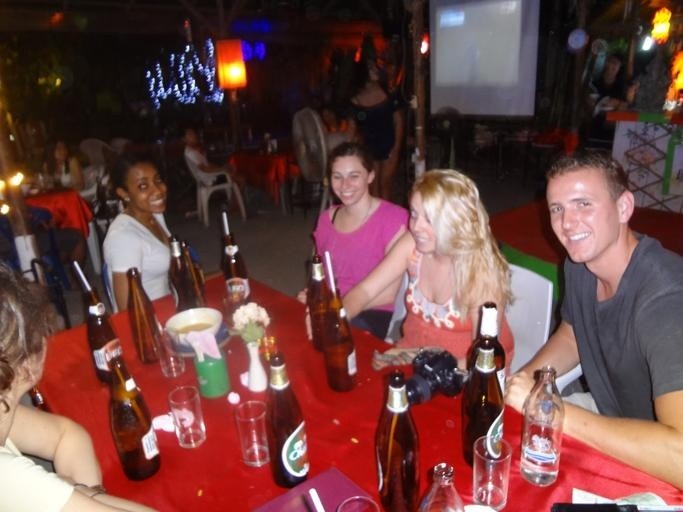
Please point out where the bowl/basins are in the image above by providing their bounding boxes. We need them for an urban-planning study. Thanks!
[166,307,222,347]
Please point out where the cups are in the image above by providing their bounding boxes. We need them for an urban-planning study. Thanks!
[472,436,514,511]
[235,399,273,468]
[149,328,186,379]
[169,387,208,451]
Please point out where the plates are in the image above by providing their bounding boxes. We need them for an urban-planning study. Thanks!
[162,323,235,355]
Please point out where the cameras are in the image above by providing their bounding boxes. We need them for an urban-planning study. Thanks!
[406,349,464,404]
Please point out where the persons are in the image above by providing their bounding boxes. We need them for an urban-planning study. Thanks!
[590,55,639,110]
[503,152,683,491]
[306,169,515,377]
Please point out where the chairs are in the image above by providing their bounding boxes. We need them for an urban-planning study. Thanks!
[502,262,553,379]
[186,148,248,225]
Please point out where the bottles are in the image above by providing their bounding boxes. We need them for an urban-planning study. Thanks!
[167,235,205,312]
[83,289,165,479]
[461,302,508,465]
[10,163,78,197]
[376,369,420,512]
[122,265,168,364]
[414,463,465,511]
[191,349,230,399]
[305,256,358,390]
[211,132,285,155]
[522,365,562,489]
[267,349,313,489]
[218,209,252,309]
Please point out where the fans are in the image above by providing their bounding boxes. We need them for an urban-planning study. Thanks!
[291,107,350,257]
[86,140,121,276]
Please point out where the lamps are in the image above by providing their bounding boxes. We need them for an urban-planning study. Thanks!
[216,37,251,93]
